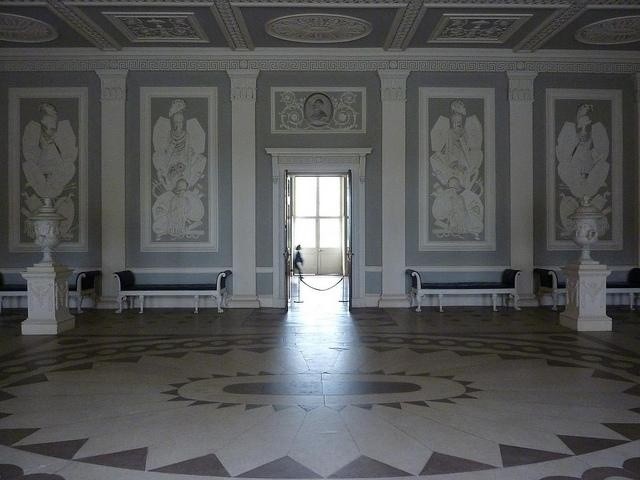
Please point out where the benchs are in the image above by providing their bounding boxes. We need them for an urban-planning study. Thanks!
[1,268,233,315]
[404,268,640,313]
